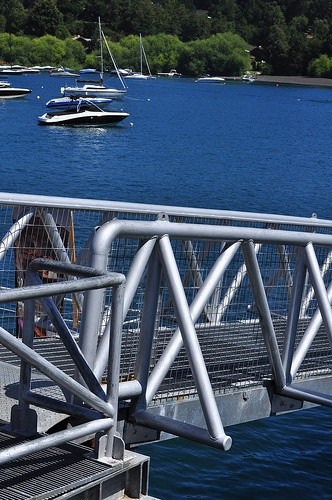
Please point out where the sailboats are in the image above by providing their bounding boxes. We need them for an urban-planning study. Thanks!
[61,30,129,99]
[124,32,157,81]
[74,16,104,87]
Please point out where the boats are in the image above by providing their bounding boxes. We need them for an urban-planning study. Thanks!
[79,68,97,74]
[0,63,81,88]
[156,69,182,77]
[36,107,129,127]
[194,74,226,84]
[37,92,114,115]
[0,88,33,99]
[109,68,135,76]
